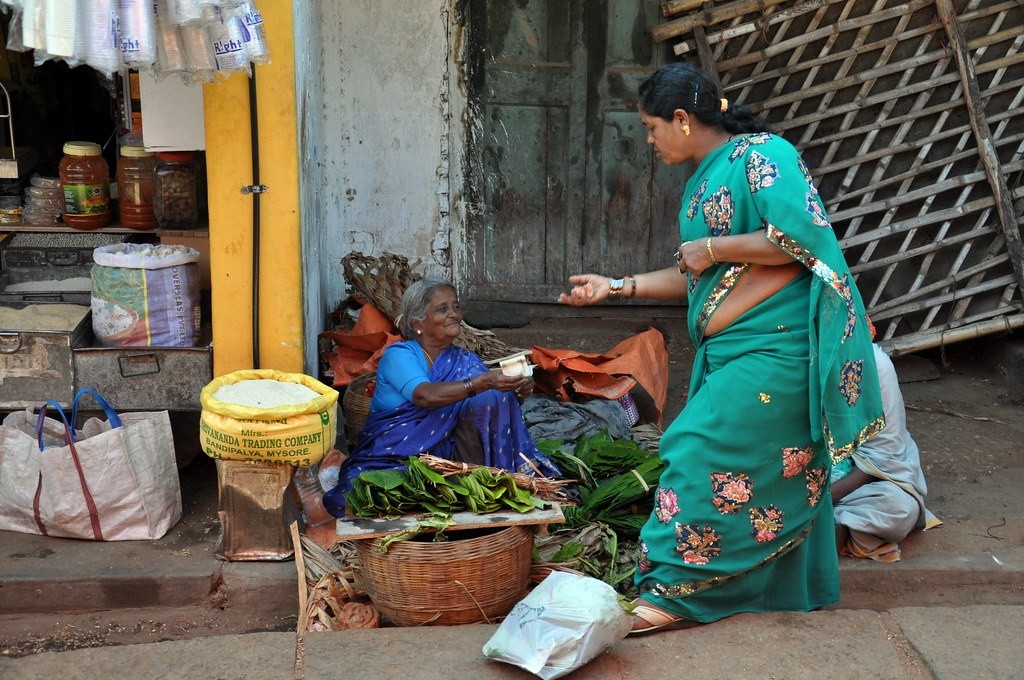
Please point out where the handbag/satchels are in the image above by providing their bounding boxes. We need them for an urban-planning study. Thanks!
[483,569,633,680]
[0,389,182,541]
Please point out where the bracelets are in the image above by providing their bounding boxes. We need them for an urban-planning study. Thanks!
[706,237,718,264]
[629,275,636,298]
[463,377,476,398]
[608,277,624,301]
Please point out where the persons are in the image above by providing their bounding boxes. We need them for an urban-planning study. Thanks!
[559,62,887,635]
[831,314,927,564]
[322,279,564,538]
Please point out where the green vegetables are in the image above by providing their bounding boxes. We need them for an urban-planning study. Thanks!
[343,428,669,614]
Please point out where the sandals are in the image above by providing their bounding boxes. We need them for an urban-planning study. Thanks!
[623,596,702,636]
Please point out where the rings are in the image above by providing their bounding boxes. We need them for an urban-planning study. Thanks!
[673,252,680,261]
[531,388,533,392]
[677,267,686,274]
[676,260,679,265]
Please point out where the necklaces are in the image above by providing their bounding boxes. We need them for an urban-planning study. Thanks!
[725,135,733,144]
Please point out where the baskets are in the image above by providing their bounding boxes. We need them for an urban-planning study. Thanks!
[355,524,534,625]
[341,372,380,447]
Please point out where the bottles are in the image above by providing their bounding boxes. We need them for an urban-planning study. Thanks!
[59,141,111,230]
[117,146,198,230]
